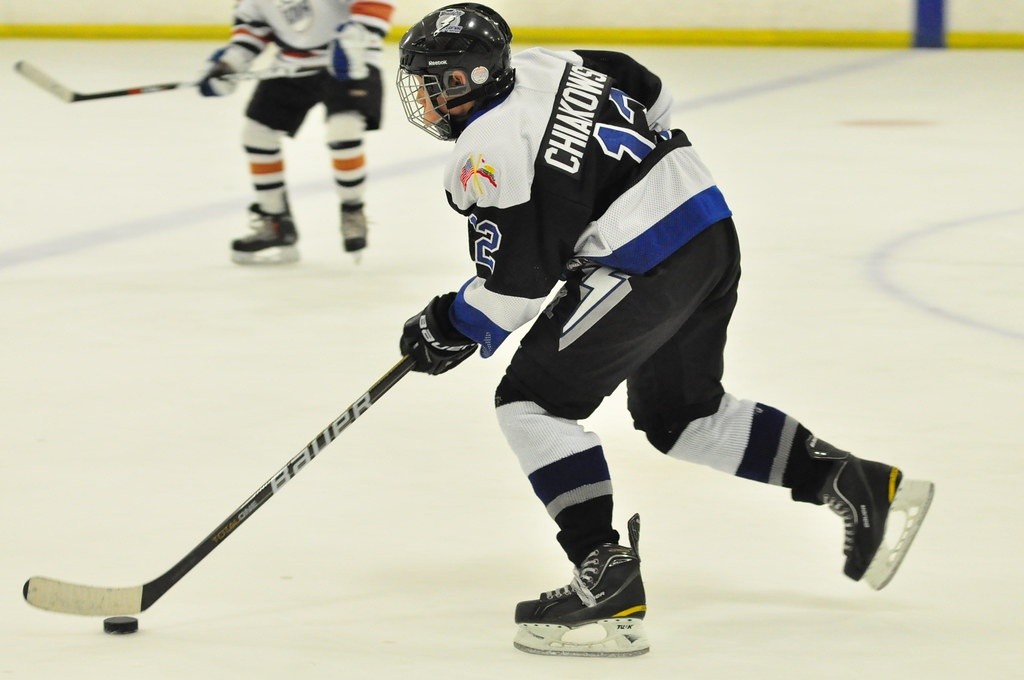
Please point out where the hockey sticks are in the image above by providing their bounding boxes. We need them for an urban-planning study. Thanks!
[19,348,424,618]
[12,58,329,105]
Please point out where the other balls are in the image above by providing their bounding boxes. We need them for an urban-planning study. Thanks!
[103,616,138,634]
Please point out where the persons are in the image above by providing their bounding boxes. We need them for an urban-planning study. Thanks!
[201,0,395,265]
[397,2,934,659]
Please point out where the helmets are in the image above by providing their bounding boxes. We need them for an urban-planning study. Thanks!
[395,2,514,141]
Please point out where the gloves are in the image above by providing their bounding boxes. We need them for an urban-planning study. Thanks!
[327,19,384,82]
[199,44,255,97]
[400,292,478,376]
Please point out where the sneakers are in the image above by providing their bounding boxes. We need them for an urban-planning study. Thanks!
[340,202,369,263]
[805,435,935,591]
[513,512,651,658]
[231,194,299,264]
